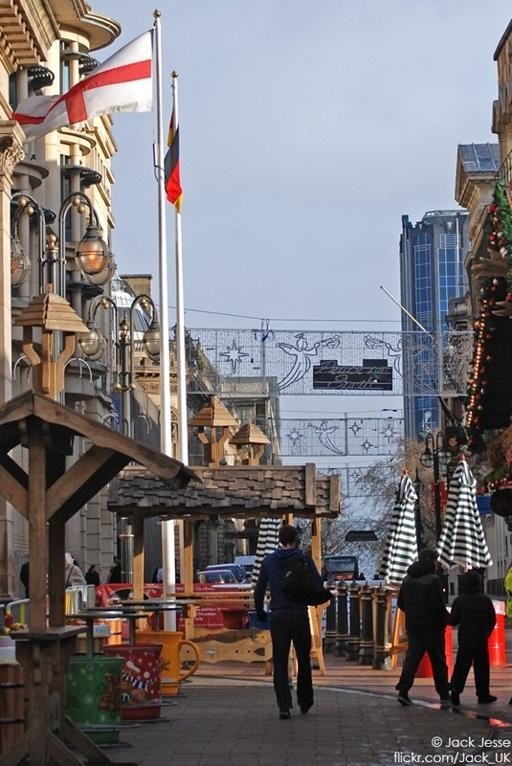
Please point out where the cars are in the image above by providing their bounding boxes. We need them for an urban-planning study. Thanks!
[191,554,257,585]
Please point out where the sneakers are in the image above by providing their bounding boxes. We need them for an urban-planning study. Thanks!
[397,693,412,705]
[440,694,460,707]
[278,709,290,718]
[477,695,497,704]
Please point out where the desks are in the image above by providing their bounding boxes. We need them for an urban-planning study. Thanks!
[59,592,198,732]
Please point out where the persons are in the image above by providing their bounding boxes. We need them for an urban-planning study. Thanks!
[442,569,500,704]
[20,561,29,600]
[108,555,121,583]
[394,547,456,708]
[65,552,87,591]
[85,565,99,586]
[253,523,323,721]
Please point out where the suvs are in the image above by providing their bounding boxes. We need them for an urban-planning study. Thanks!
[322,556,360,583]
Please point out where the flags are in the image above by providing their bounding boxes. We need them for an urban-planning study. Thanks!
[163,93,184,213]
[13,27,158,142]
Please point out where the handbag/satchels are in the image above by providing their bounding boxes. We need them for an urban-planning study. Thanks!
[277,552,332,605]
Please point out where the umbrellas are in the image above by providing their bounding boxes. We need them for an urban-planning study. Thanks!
[431,453,495,574]
[376,464,419,664]
[251,516,286,604]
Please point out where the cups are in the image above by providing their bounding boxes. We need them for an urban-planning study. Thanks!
[134,631,200,695]
[63,654,126,744]
[100,643,164,721]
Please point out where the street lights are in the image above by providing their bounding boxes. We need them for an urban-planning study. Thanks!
[418,428,452,605]
[10,188,116,403]
[76,290,162,602]
